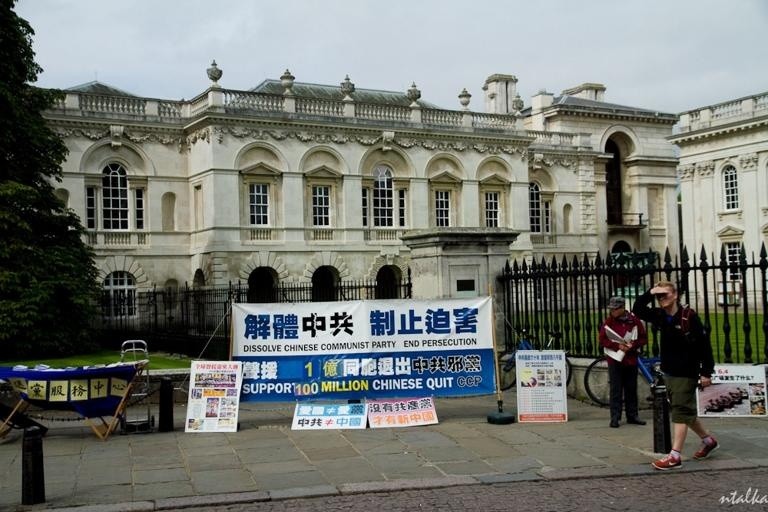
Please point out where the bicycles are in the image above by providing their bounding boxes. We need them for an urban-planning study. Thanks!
[498,329,571,391]
[585,350,673,410]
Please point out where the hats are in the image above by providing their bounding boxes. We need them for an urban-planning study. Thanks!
[608,297,625,309]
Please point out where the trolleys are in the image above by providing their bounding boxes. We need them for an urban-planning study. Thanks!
[119,340,155,435]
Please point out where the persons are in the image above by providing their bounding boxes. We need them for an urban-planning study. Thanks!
[633,281,721,470]
[599,297,648,427]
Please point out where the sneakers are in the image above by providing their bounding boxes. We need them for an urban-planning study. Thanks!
[652,454,682,470]
[610,417,619,427]
[627,417,647,425]
[694,438,719,459]
